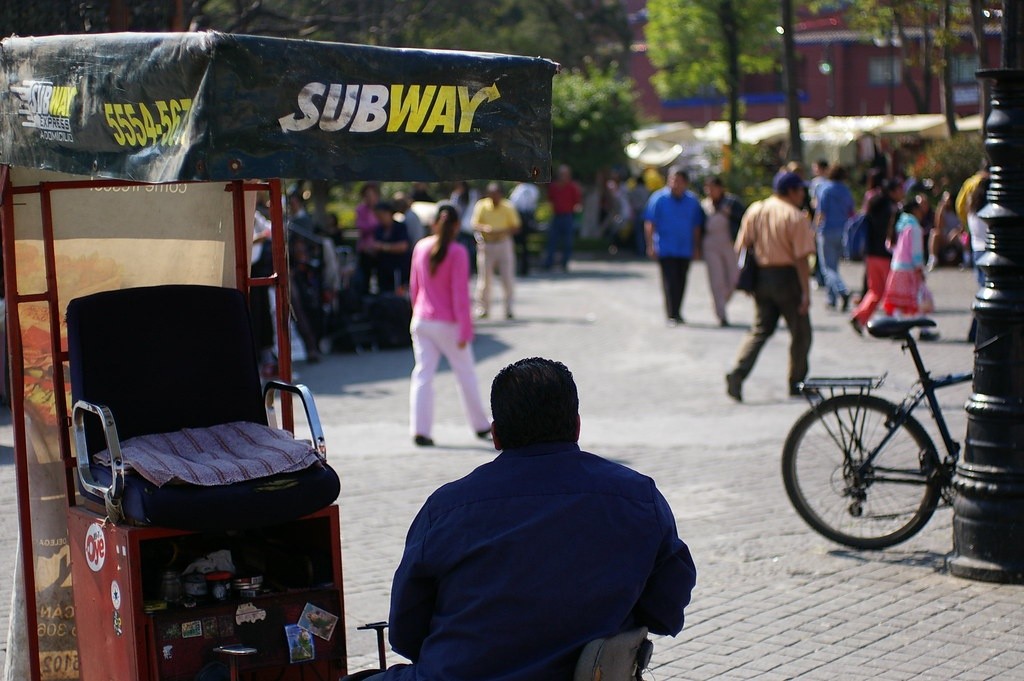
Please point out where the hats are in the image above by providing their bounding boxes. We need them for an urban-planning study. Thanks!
[776,171,812,190]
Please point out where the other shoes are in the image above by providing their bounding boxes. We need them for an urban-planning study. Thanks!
[413,434,433,446]
[477,429,492,438]
[828,301,835,308]
[842,294,850,311]
[726,374,741,400]
[918,330,938,341]
[850,317,861,334]
[790,386,820,398]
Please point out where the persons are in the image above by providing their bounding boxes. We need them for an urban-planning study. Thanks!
[407,203,492,444]
[450,182,541,321]
[600,175,648,252]
[883,199,940,340]
[644,170,702,322]
[772,161,853,311]
[545,165,583,271]
[855,161,989,304]
[353,358,697,681]
[727,173,817,398]
[700,174,744,326]
[852,176,904,334]
[250,181,439,378]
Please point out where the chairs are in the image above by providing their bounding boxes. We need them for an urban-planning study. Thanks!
[67,284,341,527]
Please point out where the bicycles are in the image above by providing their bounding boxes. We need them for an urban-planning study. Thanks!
[780,315,974,549]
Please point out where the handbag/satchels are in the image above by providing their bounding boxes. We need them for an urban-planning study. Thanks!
[846,214,869,262]
[733,243,760,294]
